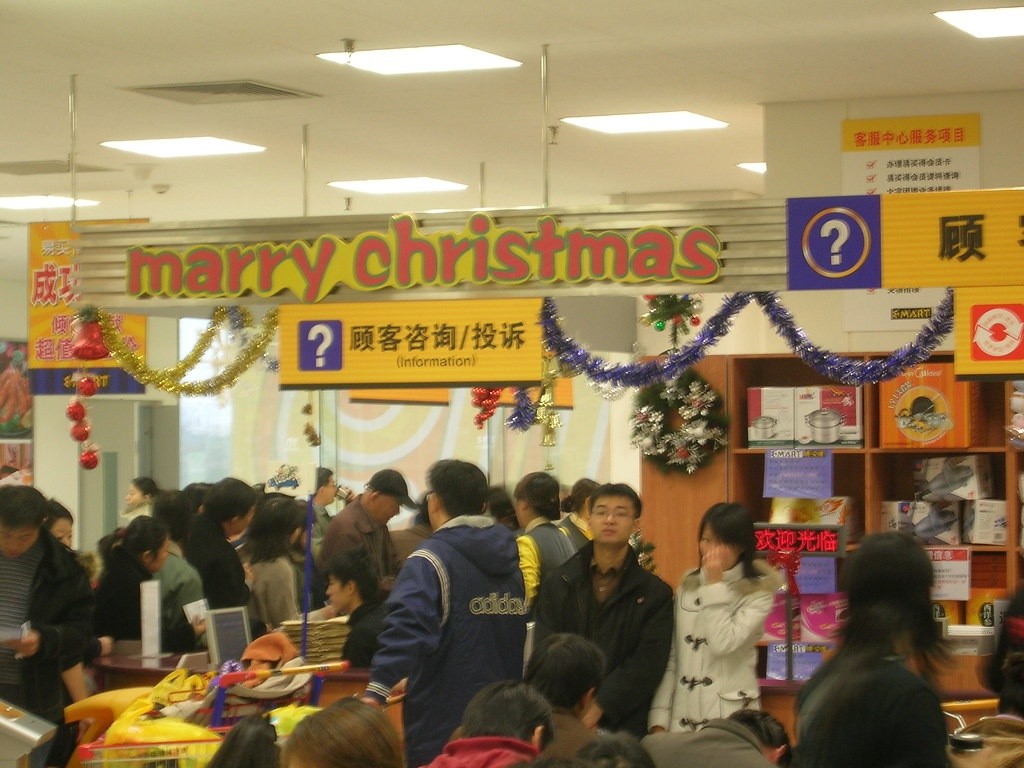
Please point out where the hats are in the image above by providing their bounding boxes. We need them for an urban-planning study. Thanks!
[367,468,417,509]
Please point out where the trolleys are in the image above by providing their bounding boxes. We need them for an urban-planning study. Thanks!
[76,658,351,768]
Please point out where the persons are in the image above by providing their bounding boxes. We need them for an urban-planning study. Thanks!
[796,531,955,768]
[977,576,1024,720]
[0,459,793,768]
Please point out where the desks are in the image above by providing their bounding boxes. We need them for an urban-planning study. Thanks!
[89,638,411,750]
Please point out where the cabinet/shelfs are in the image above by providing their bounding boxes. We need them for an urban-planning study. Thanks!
[637,350,1019,746]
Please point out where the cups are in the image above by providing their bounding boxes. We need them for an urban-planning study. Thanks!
[336,485,350,499]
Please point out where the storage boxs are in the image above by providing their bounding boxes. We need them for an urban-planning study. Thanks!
[746,359,1023,678]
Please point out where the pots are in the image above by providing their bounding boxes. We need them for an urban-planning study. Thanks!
[804,408,847,443]
[752,416,777,439]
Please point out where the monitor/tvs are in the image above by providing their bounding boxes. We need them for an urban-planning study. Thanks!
[204,605,253,665]
